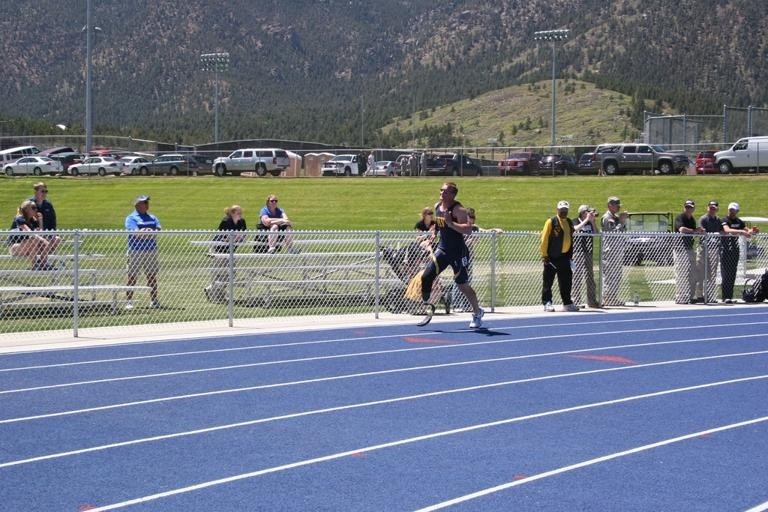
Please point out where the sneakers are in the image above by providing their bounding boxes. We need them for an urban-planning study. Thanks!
[732,299,737,304]
[205,286,213,301]
[564,303,579,311]
[291,247,301,253]
[41,190,47,192]
[268,246,276,253]
[724,299,731,303]
[575,304,585,308]
[469,308,484,328]
[589,303,604,308]
[543,301,554,311]
[698,297,704,302]
[150,300,161,308]
[124,303,133,310]
[692,299,698,303]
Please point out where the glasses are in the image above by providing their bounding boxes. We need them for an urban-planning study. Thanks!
[268,200,278,203]
[31,206,37,209]
[440,189,447,193]
[425,213,434,214]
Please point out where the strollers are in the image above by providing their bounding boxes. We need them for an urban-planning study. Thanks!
[377,241,455,315]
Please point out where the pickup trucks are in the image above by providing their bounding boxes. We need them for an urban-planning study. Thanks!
[426,154,483,176]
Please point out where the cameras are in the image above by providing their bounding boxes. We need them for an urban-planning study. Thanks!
[588,207,599,218]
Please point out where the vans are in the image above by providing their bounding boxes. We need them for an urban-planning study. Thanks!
[0,145,41,174]
[713,135,768,172]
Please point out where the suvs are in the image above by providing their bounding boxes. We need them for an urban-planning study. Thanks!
[211,147,291,177]
[695,149,722,173]
[137,153,198,176]
[537,154,575,176]
[590,143,689,174]
[320,154,363,177]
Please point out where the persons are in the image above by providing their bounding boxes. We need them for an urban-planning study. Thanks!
[124,195,162,309]
[204,205,247,302]
[696,200,723,303]
[414,206,435,231]
[672,200,706,304]
[28,181,57,237]
[363,151,376,178]
[720,202,753,303]
[572,205,604,308]
[400,150,427,176]
[7,200,58,270]
[601,195,629,306]
[539,200,580,312]
[253,194,301,254]
[416,182,484,328]
[452,207,503,313]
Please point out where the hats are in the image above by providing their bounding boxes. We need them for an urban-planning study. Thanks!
[684,200,695,208]
[133,195,151,207]
[728,202,740,211]
[609,196,623,206]
[578,205,590,212]
[466,208,475,219]
[557,200,569,209]
[707,200,718,206]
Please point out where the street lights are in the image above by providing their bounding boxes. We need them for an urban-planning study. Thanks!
[200,52,230,143]
[534,29,571,146]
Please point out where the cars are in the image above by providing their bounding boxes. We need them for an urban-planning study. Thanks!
[497,151,540,176]
[119,155,149,175]
[2,156,64,176]
[66,156,123,176]
[365,160,397,177]
